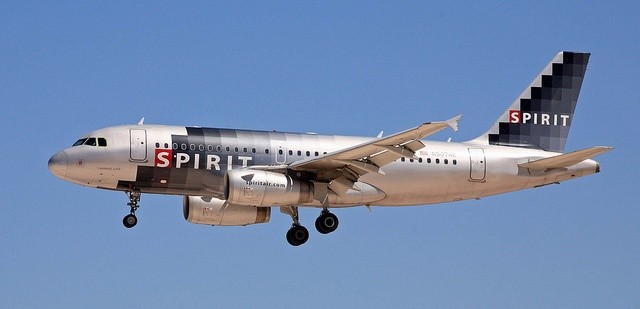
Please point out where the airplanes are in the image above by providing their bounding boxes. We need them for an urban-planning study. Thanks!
[49,51,615,246]
